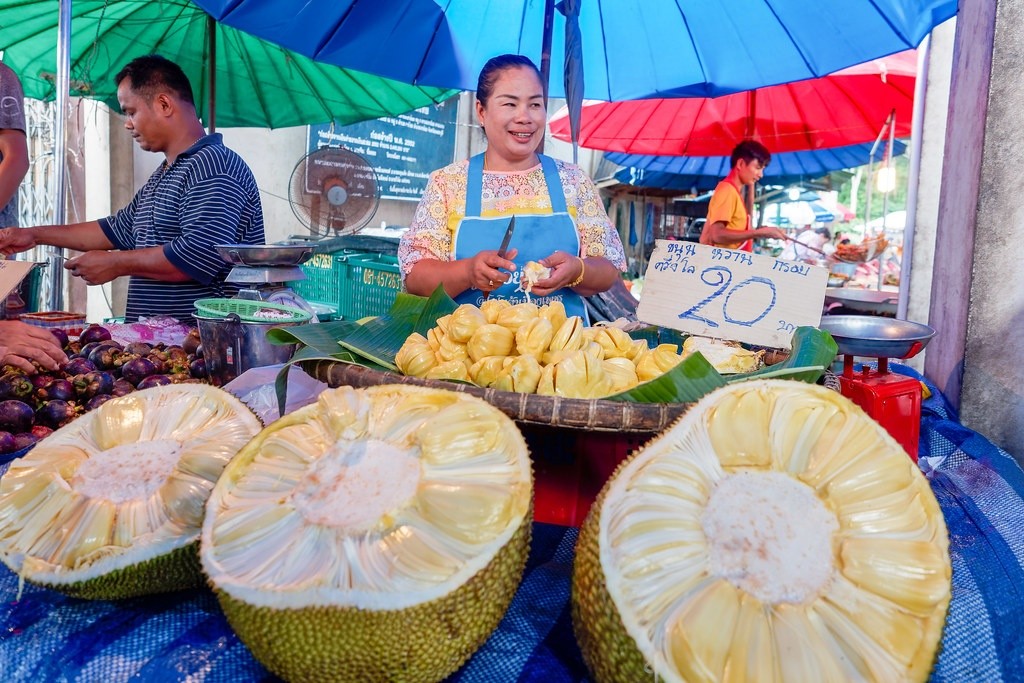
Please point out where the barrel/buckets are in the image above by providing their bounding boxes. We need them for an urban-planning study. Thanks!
[192,310,298,387]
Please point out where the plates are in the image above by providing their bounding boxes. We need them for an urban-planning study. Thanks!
[213,244,319,265]
[818,314,938,358]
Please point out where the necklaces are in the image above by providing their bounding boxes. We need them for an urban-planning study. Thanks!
[485,153,488,171]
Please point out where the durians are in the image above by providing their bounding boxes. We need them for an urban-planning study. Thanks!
[199,383,534,683]
[570,377,951,683]
[0,383,265,598]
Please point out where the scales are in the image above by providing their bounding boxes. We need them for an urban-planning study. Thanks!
[212,245,336,320]
[821,316,936,467]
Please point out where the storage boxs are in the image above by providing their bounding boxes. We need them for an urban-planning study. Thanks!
[286,250,363,306]
[335,252,402,322]
[19,310,87,341]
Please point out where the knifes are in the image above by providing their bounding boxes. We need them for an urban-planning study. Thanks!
[482,213,515,298]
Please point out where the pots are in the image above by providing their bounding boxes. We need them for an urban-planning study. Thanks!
[823,287,899,314]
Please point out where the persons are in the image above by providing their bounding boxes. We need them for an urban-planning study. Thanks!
[0,63,30,261]
[700,138,787,253]
[397,56,628,328]
[832,232,841,251]
[776,227,831,264]
[0,56,265,326]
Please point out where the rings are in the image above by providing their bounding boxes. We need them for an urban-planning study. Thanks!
[489,280,493,287]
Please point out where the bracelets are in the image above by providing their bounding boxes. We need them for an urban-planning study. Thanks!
[562,256,585,288]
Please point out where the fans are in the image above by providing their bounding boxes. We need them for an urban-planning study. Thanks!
[287,145,381,242]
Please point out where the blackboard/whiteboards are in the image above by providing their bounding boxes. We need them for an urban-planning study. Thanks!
[634,239,829,362]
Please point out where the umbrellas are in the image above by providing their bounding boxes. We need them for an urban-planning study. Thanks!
[0,0,961,312]
